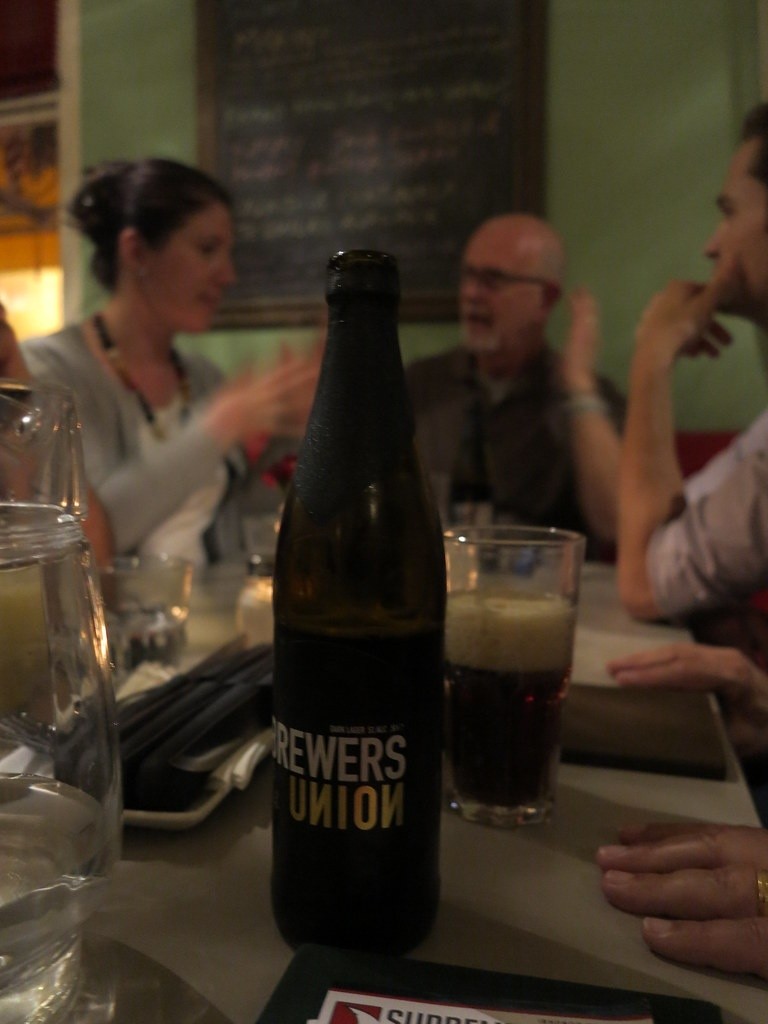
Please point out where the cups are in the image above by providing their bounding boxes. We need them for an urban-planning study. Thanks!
[444,525,602,824]
[111,555,194,650]
[235,555,274,650]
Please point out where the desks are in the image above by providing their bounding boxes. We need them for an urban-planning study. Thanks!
[0,562,768,1024]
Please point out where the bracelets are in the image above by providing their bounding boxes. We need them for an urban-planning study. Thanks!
[565,384,598,410]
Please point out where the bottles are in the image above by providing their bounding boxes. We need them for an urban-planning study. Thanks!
[268,250,448,956]
[0,377,123,1024]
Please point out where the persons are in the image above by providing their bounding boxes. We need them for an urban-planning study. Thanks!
[24,155,324,559]
[411,214,622,566]
[0,306,116,574]
[595,645,768,982]
[617,100,767,627]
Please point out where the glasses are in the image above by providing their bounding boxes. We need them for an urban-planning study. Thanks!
[451,261,551,290]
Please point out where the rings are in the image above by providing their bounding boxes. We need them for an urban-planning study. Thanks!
[756,871,768,914]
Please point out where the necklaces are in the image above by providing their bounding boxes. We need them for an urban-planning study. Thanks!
[95,315,191,441]
